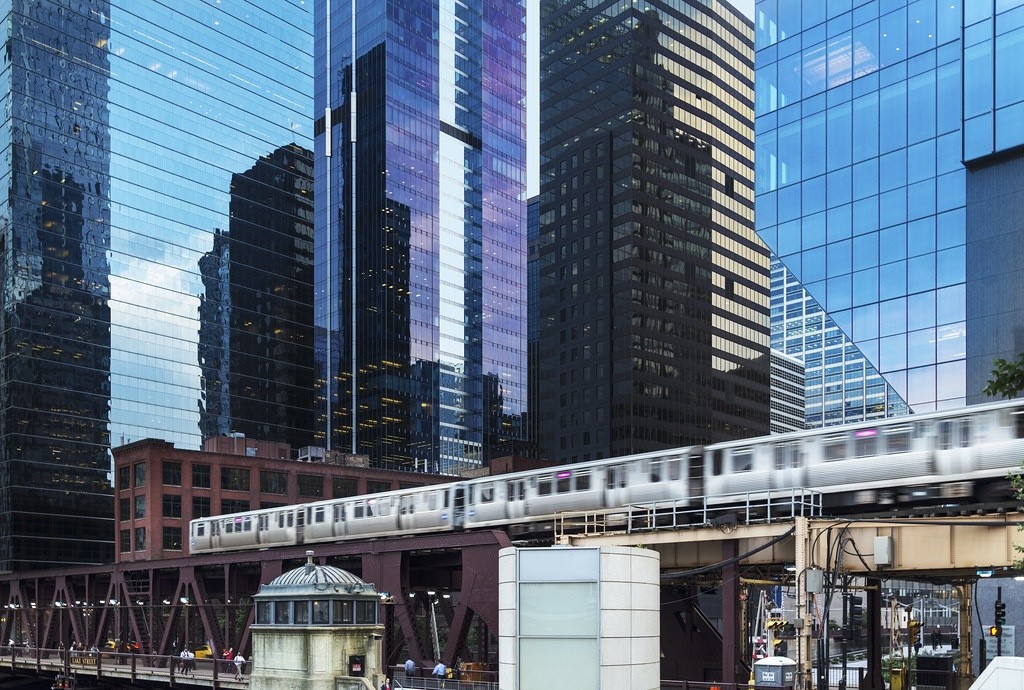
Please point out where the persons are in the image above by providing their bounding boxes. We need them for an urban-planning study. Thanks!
[68,641,78,657]
[937,630,943,648]
[234,651,246,680]
[248,653,253,672]
[381,677,392,690]
[188,650,195,672]
[223,647,234,673]
[179,647,190,676]
[88,644,100,658]
[7,638,16,650]
[404,656,416,688]
[77,642,86,657]
[56,640,67,664]
[431,659,446,690]
[914,641,919,654]
[930,630,938,650]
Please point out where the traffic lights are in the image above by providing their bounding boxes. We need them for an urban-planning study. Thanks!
[908,620,925,629]
[912,628,920,644]
[765,618,789,630]
[773,638,784,646]
[989,626,999,637]
[994,600,1006,626]
[848,594,864,628]
